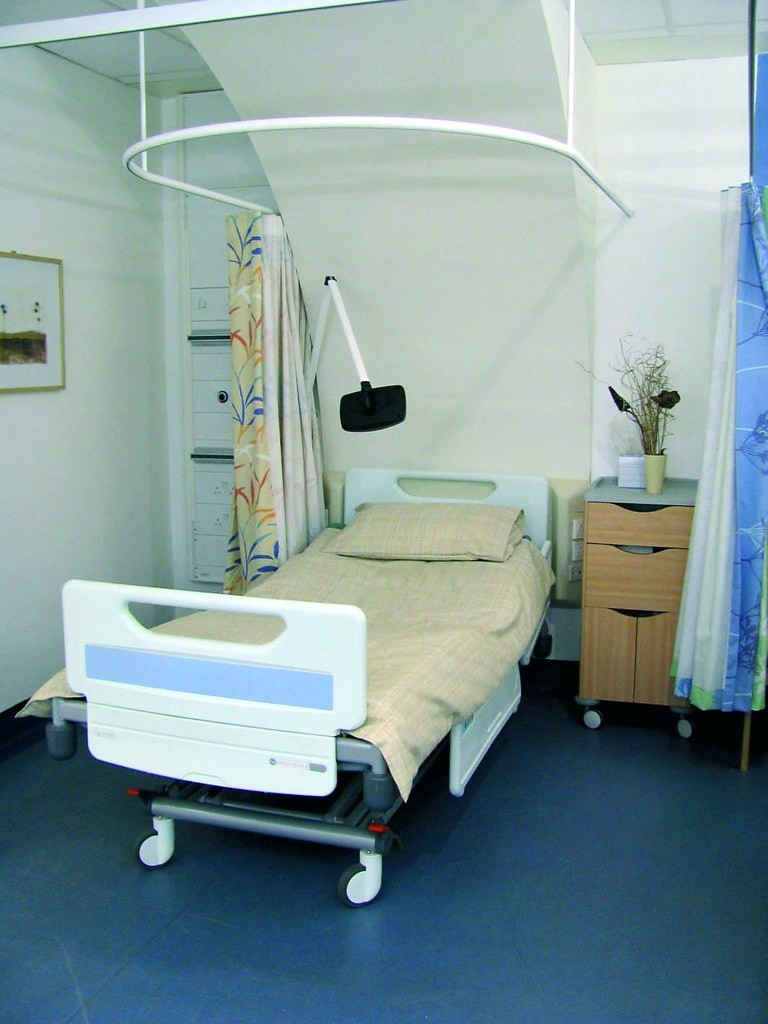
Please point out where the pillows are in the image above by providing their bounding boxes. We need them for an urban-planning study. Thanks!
[324,501,527,562]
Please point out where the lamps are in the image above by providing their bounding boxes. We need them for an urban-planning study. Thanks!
[307,276,407,434]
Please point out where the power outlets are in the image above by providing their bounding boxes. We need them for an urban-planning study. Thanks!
[573,519,584,539]
[568,565,582,582]
[572,542,584,562]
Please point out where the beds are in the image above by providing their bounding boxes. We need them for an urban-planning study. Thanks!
[15,468,557,905]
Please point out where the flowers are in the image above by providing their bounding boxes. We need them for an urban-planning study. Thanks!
[574,331,681,455]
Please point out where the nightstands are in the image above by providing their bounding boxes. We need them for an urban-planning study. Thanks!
[575,475,699,736]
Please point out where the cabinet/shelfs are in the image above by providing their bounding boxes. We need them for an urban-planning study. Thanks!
[175,89,282,585]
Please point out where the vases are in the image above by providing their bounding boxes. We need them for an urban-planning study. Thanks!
[644,455,666,495]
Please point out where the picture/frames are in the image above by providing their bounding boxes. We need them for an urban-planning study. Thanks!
[0,249,67,394]
